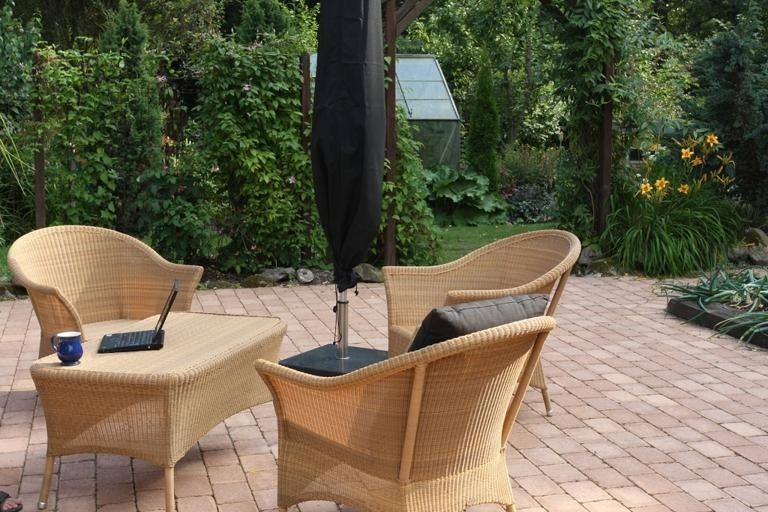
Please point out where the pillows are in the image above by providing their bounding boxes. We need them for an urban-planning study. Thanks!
[409,293,549,352]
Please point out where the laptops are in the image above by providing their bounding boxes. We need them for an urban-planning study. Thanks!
[97,277,181,354]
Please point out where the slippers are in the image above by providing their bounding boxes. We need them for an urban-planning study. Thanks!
[0,491,24,512]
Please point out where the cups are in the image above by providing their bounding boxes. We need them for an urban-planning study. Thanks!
[51,331,83,366]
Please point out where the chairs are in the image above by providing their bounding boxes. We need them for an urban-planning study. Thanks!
[254,313,555,512]
[4,224,205,357]
[377,228,582,444]
